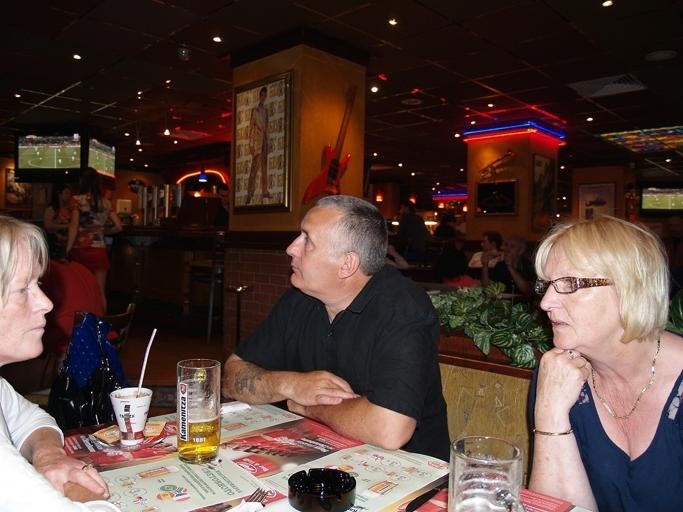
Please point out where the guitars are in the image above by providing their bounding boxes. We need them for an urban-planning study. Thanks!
[303,84,362,204]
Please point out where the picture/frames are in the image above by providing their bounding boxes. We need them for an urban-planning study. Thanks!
[231,69,294,214]
[5,167,33,207]
[532,152,556,234]
[577,182,616,223]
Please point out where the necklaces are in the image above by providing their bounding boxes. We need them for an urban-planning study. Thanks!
[587,333,661,420]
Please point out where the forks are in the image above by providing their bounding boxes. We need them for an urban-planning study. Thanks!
[244,487,269,503]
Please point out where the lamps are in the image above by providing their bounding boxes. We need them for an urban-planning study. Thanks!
[199,165,208,183]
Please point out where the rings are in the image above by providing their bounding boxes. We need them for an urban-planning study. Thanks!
[88,463,93,470]
[565,349,574,360]
[81,465,87,470]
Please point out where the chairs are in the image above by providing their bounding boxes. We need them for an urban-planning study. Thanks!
[41,302,136,386]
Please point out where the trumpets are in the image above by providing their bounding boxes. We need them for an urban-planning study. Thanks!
[476,145,515,180]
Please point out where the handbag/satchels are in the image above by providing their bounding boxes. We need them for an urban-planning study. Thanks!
[49,312,122,429]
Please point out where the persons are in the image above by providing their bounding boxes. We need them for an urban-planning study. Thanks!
[397,201,427,242]
[244,87,273,204]
[221,193,451,512]
[63,165,121,317]
[44,185,72,232]
[434,214,455,236]
[0,216,118,512]
[526,212,683,512]
[467,231,503,282]
[385,242,410,271]
[480,233,535,299]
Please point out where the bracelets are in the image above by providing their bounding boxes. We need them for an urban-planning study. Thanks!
[532,429,575,436]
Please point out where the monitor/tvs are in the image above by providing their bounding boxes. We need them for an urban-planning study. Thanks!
[88,138,116,178]
[17,135,81,169]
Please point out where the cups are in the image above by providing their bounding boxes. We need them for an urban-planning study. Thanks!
[447,435,526,512]
[176,358,223,465]
[109,386,154,447]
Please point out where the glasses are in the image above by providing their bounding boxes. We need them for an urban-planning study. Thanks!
[534,276,615,294]
